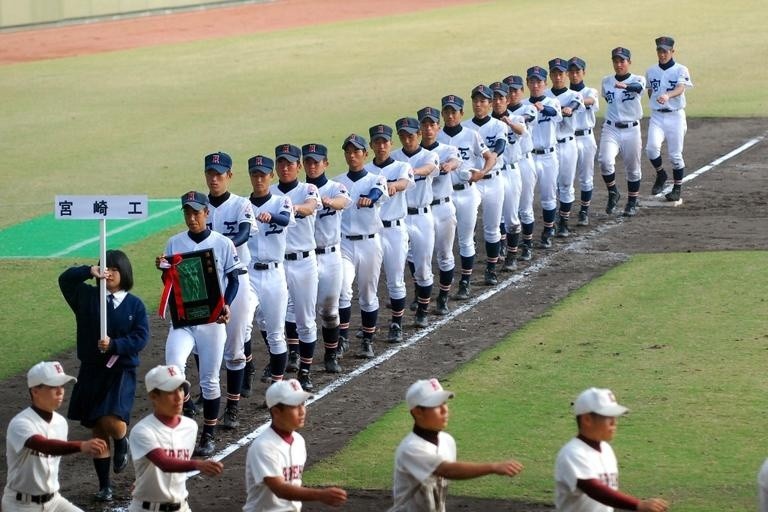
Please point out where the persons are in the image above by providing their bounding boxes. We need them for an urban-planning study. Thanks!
[596,47,646,216]
[334,94,497,360]
[646,37,695,201]
[382,375,526,511]
[239,377,351,512]
[550,384,673,512]
[153,143,351,456]
[54,248,151,503]
[124,363,226,511]
[0,362,110,511]
[463,58,599,285]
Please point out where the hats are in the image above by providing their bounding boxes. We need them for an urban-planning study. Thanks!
[471,57,585,98]
[575,388,628,417]
[612,48,630,61]
[656,37,674,51]
[407,378,453,410]
[266,378,313,408]
[26,360,78,388]
[145,365,186,393]
[179,191,207,210]
[206,96,462,175]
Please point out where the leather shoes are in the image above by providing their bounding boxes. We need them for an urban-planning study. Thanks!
[97,487,112,500]
[113,437,129,473]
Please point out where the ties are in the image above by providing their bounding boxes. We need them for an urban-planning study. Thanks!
[108,294,114,309]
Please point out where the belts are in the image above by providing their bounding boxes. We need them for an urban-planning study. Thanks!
[143,502,180,512]
[241,198,451,273]
[16,492,54,504]
[657,109,671,112]
[608,121,637,127]
[453,128,591,190]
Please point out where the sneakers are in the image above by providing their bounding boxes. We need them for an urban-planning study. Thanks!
[182,366,256,457]
[261,301,449,389]
[606,173,680,216]
[454,211,589,300]
